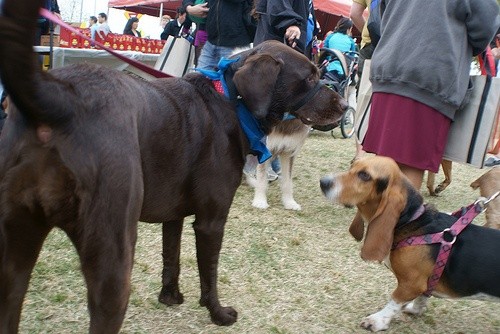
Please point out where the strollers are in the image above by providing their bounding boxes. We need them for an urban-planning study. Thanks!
[315,47,360,139]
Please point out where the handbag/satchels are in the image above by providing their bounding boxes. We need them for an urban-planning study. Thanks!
[443,51,499,169]
[154,20,198,79]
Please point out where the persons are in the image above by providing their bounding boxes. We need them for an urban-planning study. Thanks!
[323,17,357,93]
[150,14,171,41]
[350,0,376,152]
[180,0,258,79]
[123,16,142,38]
[89,16,98,41]
[367,1,500,196]
[253,0,319,55]
[160,6,187,40]
[97,11,111,40]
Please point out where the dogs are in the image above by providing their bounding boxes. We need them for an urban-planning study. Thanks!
[0,0,348,334]
[319,139,500,333]
[470,164,500,231]
[227,45,311,211]
